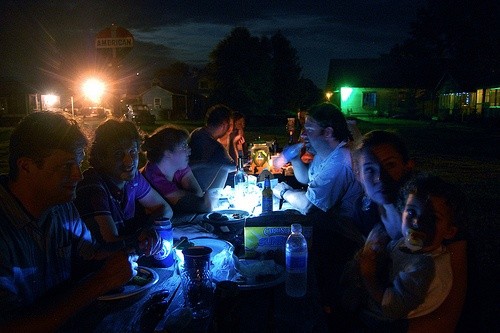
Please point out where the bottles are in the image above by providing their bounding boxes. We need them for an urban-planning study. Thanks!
[286,223,308,297]
[234,166,245,186]
[261,175,273,212]
[269,141,305,170]
[238,158,245,183]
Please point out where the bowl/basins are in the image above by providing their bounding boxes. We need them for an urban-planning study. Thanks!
[202,209,251,238]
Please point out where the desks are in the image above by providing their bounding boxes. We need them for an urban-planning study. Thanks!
[64,150,317,333]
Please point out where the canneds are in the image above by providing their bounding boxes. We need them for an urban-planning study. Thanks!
[151,218,173,268]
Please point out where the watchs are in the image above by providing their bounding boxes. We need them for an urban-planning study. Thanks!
[280,188,292,200]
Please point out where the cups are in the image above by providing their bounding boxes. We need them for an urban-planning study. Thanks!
[182,269,214,318]
[178,259,209,289]
[182,246,213,274]
[150,216,173,268]
[288,118,296,130]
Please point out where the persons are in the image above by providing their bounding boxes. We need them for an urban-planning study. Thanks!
[272,102,366,233]
[139,124,211,214]
[187,104,249,191]
[73,120,174,242]
[0,111,139,333]
[323,130,466,333]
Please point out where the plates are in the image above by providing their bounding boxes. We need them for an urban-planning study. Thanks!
[77,266,159,299]
[228,259,287,289]
